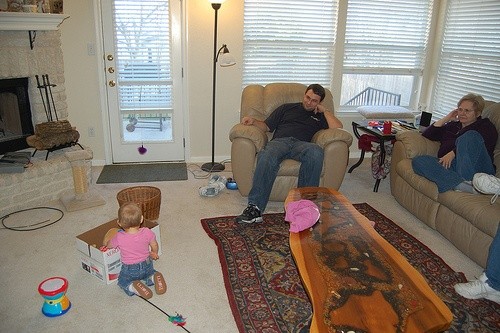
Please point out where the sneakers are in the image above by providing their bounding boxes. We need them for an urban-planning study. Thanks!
[454,272,500,303]
[472,173,500,204]
[235,204,263,224]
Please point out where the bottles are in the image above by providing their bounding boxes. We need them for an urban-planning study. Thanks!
[384,119,392,134]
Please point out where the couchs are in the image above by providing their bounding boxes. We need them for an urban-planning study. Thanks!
[229,82,353,202]
[390,100,500,268]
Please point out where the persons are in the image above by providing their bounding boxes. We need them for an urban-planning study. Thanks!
[453,223,500,304]
[236,84,343,224]
[411,93,500,204]
[107,201,167,299]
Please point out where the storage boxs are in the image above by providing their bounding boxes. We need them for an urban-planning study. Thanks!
[76,219,162,286]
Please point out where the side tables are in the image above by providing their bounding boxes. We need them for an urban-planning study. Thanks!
[348,121,391,192]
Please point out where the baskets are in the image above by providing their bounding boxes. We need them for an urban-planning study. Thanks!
[117,187,161,221]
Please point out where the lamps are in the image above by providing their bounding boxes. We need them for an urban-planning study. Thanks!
[201,0,236,173]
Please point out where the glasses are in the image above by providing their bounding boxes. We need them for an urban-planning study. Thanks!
[457,108,475,114]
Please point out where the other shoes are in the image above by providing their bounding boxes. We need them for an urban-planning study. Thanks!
[131,282,152,299]
[151,273,167,295]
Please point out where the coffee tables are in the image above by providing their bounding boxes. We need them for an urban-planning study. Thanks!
[284,187,453,333]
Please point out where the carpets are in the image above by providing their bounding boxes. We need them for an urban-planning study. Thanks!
[200,202,500,333]
[96,163,188,184]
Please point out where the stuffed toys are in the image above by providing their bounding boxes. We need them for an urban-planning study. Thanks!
[100,228,124,252]
